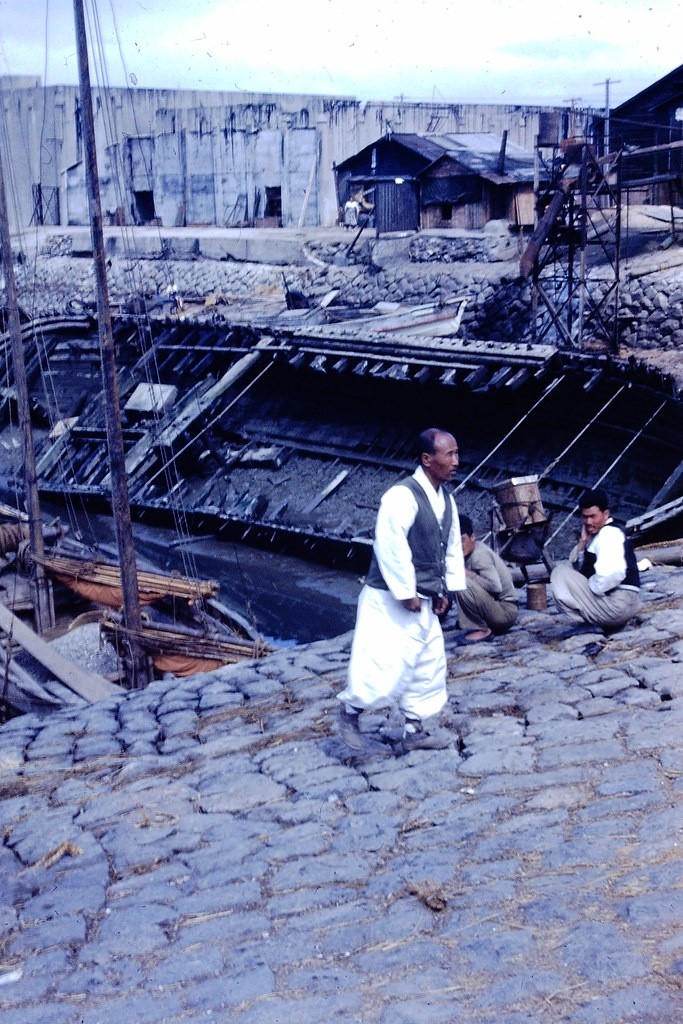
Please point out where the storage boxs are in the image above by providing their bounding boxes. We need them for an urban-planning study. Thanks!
[494,475,547,529]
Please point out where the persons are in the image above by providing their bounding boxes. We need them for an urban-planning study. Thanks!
[336,427,468,754]
[455,514,519,652]
[343,196,360,233]
[549,489,641,633]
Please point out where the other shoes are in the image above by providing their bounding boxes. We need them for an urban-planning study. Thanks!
[336,702,363,751]
[401,724,454,751]
[561,623,603,638]
[457,629,495,646]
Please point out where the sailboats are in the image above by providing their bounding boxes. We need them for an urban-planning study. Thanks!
[0,0,682,711]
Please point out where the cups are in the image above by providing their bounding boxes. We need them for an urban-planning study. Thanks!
[526,583,547,610]
